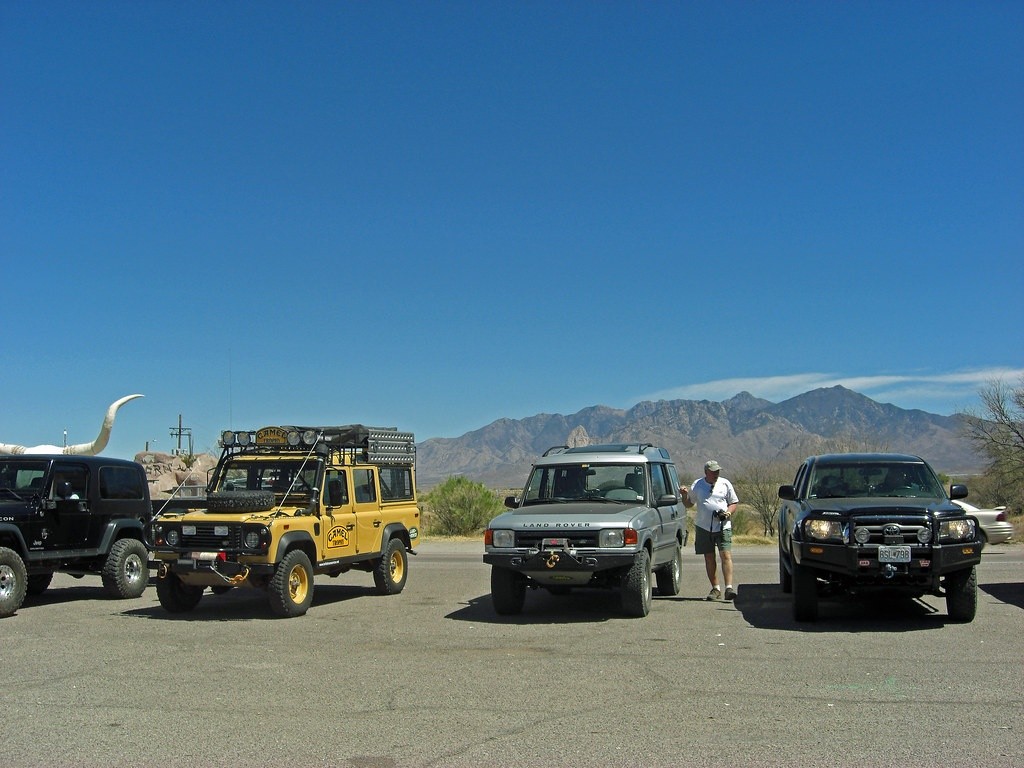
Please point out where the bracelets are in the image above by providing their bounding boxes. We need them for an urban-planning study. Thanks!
[727,512,732,516]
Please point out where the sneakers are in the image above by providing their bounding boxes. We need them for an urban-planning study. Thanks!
[725,588,737,600]
[706,588,721,600]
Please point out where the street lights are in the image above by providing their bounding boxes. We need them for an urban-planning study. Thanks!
[206,466,224,491]
[146,440,157,451]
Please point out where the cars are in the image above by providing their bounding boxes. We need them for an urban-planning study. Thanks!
[949,498,1014,546]
[149,498,209,569]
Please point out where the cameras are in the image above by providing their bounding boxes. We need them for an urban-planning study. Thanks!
[714,509,727,521]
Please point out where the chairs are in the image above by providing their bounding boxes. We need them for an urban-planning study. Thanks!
[880,471,907,494]
[327,478,345,506]
[22,477,44,495]
[625,473,644,498]
[813,476,849,497]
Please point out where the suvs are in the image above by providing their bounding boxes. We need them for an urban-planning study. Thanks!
[777,451,983,624]
[142,425,421,618]
[1,456,153,616]
[481,445,689,616]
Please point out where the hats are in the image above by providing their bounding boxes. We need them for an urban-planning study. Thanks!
[704,460,723,471]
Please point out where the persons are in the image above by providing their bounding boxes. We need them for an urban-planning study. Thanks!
[679,460,740,600]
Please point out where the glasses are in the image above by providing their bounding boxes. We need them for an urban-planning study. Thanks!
[709,484,714,496]
